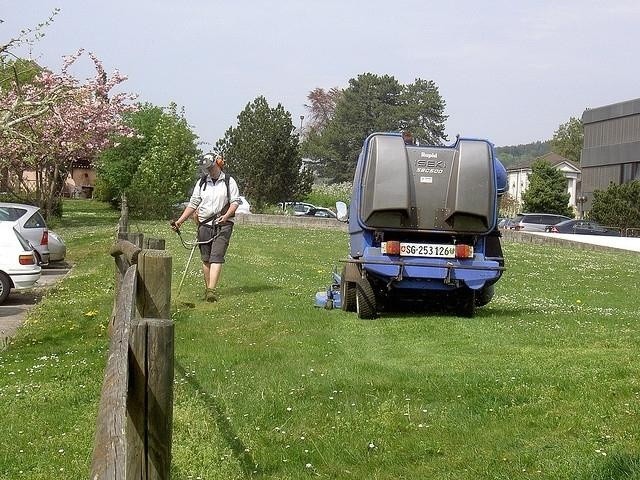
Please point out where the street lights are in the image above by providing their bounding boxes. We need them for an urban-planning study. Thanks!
[300,115,304,138]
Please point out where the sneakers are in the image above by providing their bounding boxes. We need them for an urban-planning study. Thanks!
[207,288,217,303]
[199,292,206,301]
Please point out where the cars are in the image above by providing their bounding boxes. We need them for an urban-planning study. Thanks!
[171,195,337,218]
[497,213,621,236]
[0,200,66,305]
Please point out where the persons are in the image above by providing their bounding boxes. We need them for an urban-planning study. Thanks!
[171,154,243,302]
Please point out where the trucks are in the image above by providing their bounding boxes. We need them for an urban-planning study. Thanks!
[339,132,512,319]
